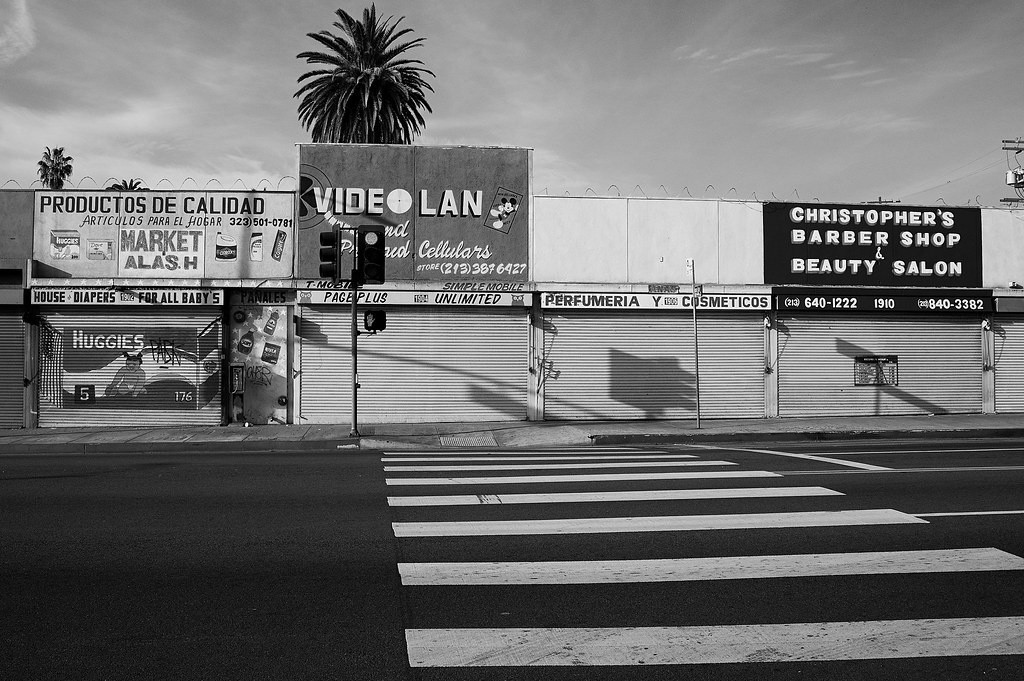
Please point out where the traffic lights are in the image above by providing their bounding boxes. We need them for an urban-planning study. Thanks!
[365,310,387,331]
[319,225,338,285]
[358,225,385,285]
